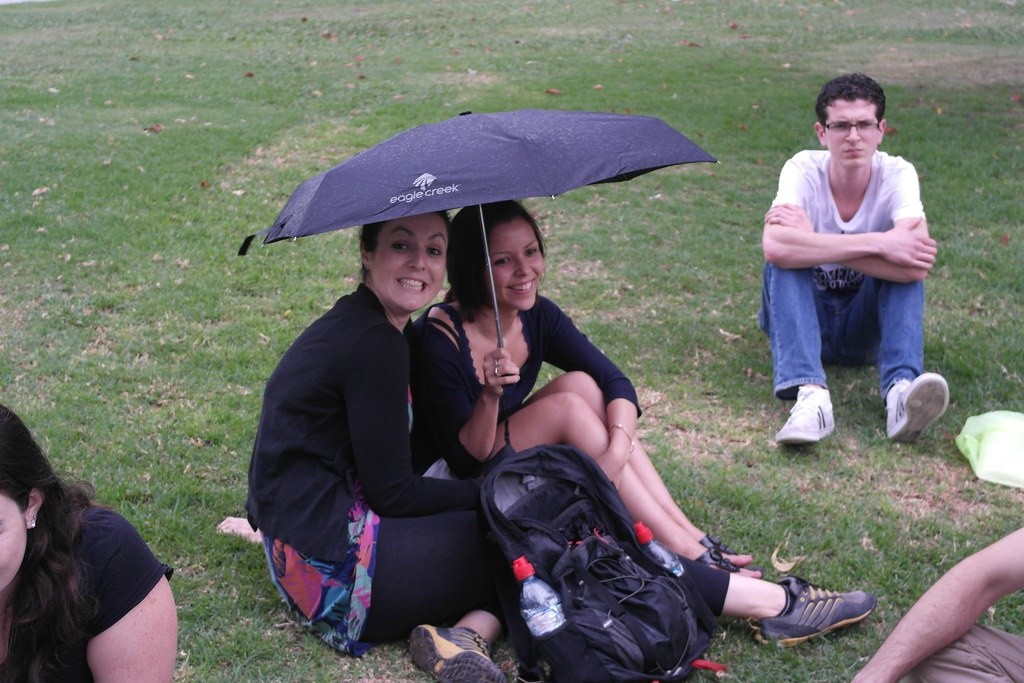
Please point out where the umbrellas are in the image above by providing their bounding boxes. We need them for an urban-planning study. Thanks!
[238,110,720,386]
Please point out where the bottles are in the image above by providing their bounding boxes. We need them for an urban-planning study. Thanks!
[513,557,561,635]
[635,522,683,577]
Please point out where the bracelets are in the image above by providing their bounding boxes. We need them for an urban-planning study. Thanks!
[610,424,634,453]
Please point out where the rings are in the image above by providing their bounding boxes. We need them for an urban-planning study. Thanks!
[494,368,500,376]
[495,358,499,367]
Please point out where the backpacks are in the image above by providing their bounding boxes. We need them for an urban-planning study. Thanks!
[476,442,724,683]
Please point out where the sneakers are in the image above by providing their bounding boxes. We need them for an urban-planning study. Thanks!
[884,373,949,441]
[757,575,875,645]
[409,623,508,682]
[776,384,835,443]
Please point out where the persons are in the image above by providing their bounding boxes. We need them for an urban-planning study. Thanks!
[408,199,764,579]
[852,526,1023,683]
[0,405,178,683]
[245,210,875,682]
[758,72,950,443]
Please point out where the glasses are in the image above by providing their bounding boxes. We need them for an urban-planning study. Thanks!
[822,118,879,131]
